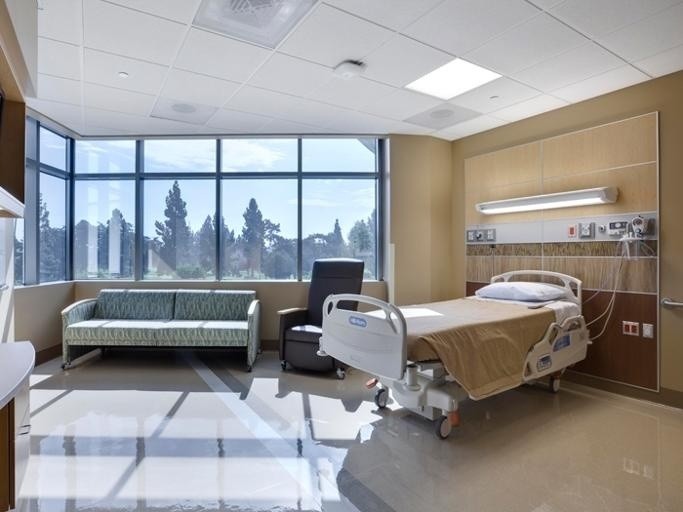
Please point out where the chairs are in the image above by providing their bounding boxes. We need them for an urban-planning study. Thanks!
[275,256,364,380]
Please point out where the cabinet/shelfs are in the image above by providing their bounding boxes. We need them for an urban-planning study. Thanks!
[0,373,32,512]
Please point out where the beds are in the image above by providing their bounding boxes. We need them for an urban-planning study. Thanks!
[317,271,592,441]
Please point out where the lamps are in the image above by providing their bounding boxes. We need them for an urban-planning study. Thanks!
[471,185,619,216]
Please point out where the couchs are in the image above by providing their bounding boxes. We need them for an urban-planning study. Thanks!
[58,287,262,374]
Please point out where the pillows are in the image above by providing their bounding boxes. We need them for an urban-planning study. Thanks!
[475,281,567,302]
